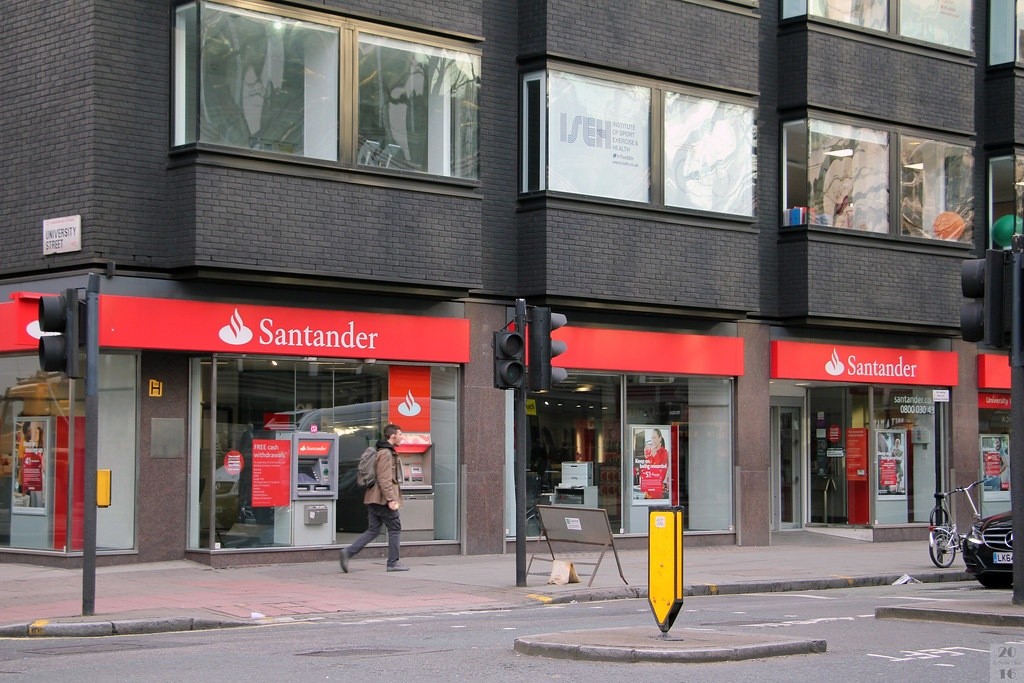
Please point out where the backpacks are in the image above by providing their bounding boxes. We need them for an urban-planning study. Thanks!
[357,446,379,489]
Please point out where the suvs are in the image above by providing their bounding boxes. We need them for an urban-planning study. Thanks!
[215,396,460,535]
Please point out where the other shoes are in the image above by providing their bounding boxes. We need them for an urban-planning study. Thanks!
[340,549,349,573]
[387,561,410,572]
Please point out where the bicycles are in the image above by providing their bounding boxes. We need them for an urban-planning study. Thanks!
[928,479,988,568]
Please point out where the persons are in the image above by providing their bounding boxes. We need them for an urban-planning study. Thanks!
[891,439,904,457]
[639,428,669,500]
[994,438,1007,491]
[530,427,559,461]
[17,421,44,495]
[895,460,903,492]
[340,424,411,573]
[240,422,256,450]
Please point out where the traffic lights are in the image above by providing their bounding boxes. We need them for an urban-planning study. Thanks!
[530,306,566,391]
[37,286,80,380]
[959,249,1010,347]
[492,331,526,389]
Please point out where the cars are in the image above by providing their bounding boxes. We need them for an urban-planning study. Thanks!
[963,508,1013,588]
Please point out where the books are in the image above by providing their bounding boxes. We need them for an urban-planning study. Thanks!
[789,208,802,225]
